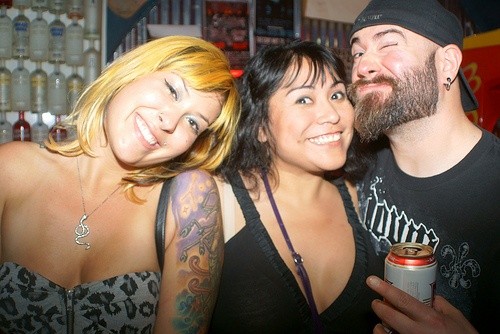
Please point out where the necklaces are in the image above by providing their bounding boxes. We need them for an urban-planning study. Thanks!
[75,158,124,251]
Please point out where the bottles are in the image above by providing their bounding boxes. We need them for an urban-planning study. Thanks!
[13,0,32,8]
[48,12,66,64]
[84,39,99,85]
[30,60,47,113]
[30,113,49,145]
[47,59,67,115]
[50,0,67,14]
[66,65,84,116]
[12,6,29,59]
[0,57,11,111]
[29,9,49,61]
[65,16,84,66]
[12,111,30,142]
[10,54,30,111]
[0,111,12,144]
[84,0,101,39]
[0,7,12,60]
[31,0,48,11]
[49,114,68,146]
[0,0,13,9]
[67,0,84,19]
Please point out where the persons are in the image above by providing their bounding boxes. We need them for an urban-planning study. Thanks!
[346,0,500,334]
[208,41,368,334]
[0,36,243,334]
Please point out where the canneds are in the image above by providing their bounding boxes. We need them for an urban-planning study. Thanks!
[382,242,437,334]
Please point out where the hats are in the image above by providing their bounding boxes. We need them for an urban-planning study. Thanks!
[348,0,477,112]
